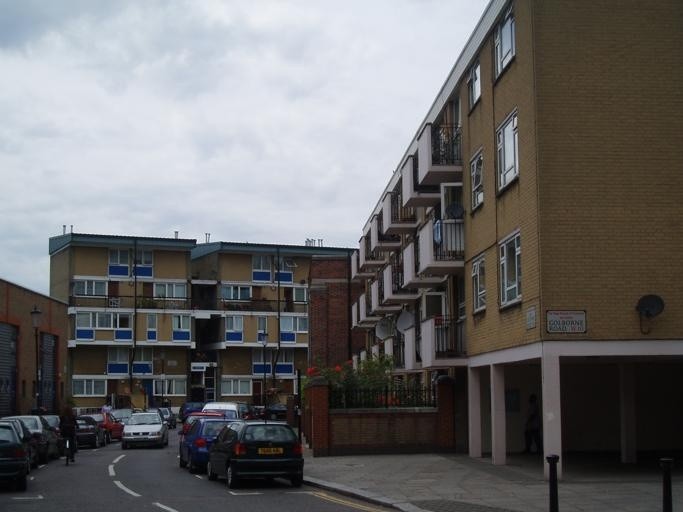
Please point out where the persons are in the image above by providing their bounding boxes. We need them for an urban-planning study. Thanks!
[59,404,79,466]
[101,401,112,414]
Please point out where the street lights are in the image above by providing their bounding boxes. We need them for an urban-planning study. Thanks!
[29,304,42,414]
[259,328,268,423]
[158,348,166,407]
